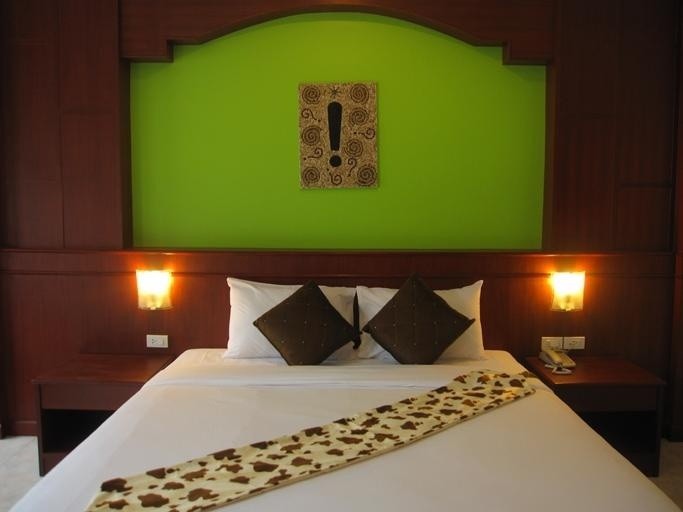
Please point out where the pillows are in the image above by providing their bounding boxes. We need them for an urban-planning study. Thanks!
[221,275,487,367]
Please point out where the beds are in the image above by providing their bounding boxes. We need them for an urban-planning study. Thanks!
[9,346,682,511]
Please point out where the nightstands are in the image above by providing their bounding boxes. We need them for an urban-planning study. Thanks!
[29,353,176,477]
[527,355,667,477]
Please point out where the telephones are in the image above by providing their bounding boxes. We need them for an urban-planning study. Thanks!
[539,349,577,368]
